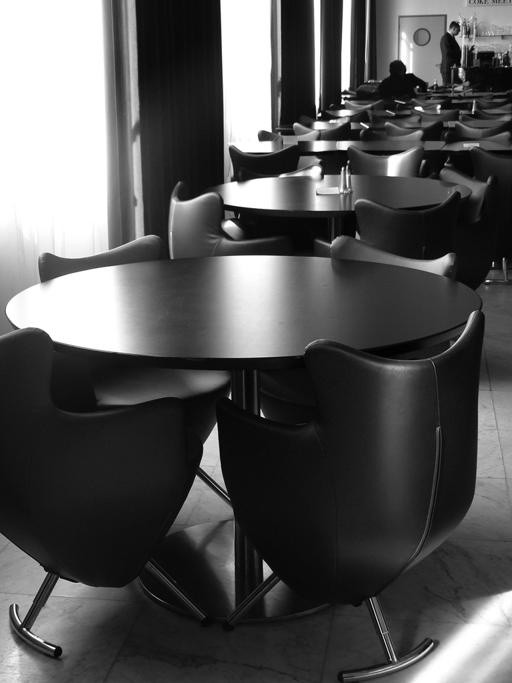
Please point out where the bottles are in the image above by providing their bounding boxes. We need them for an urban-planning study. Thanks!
[433,80,438,91]
[339,167,349,194]
[462,19,471,37]
[345,165,353,192]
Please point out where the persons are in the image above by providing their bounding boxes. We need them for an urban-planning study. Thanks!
[371,59,429,101]
[440,21,463,86]
